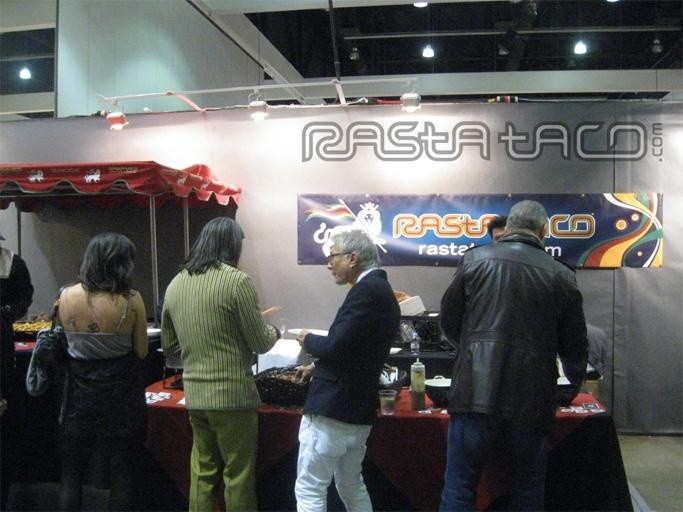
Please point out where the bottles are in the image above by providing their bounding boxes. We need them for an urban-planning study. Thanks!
[410,357,426,412]
[411,332,420,355]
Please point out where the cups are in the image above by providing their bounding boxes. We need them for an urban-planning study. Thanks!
[378,389,398,416]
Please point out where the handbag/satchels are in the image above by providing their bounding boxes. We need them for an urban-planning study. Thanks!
[25,286,66,397]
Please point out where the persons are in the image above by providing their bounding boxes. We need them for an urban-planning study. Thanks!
[488,215,508,243]
[0,234,34,435]
[294,228,402,512]
[45,231,148,512]
[439,200,592,512]
[160,216,281,512]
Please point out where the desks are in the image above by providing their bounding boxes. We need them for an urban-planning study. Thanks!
[145,368,635,512]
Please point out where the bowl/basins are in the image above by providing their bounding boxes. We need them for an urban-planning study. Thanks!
[557,379,579,407]
[426,378,452,409]
[378,370,407,402]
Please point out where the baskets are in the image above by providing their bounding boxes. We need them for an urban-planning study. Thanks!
[391,310,442,352]
[253,367,309,405]
[374,368,407,397]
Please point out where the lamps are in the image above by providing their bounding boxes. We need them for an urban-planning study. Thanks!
[248,23,269,122]
[399,86,423,113]
[106,25,127,132]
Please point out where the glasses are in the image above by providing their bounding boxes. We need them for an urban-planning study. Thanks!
[328,251,352,260]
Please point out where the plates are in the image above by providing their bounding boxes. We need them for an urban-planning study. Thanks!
[389,348,401,355]
[287,329,330,337]
[146,329,162,337]
